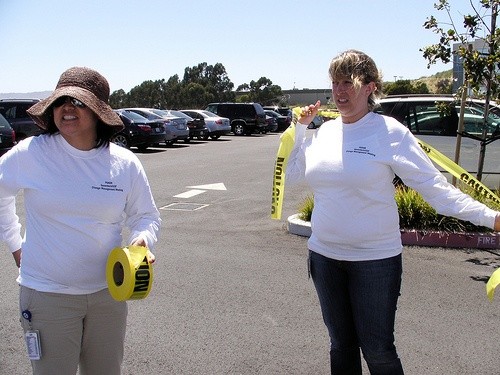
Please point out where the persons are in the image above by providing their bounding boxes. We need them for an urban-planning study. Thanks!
[0,66,161,375]
[281,49,500,375]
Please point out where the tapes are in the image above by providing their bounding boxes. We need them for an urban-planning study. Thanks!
[105,246,152,303]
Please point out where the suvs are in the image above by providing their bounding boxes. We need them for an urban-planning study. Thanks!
[263,106,292,122]
[0,98,50,143]
[372,94,500,191]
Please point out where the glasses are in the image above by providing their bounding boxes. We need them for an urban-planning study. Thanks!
[54,96,88,109]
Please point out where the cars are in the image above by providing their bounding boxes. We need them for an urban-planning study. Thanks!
[124,108,189,145]
[405,113,497,131]
[263,110,291,133]
[0,113,16,155]
[403,111,454,126]
[264,115,278,134]
[162,109,208,142]
[112,109,165,149]
[177,109,232,142]
[425,107,491,120]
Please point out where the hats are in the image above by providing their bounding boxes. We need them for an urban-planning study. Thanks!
[27,67,125,141]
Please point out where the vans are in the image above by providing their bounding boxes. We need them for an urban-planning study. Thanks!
[198,102,264,134]
